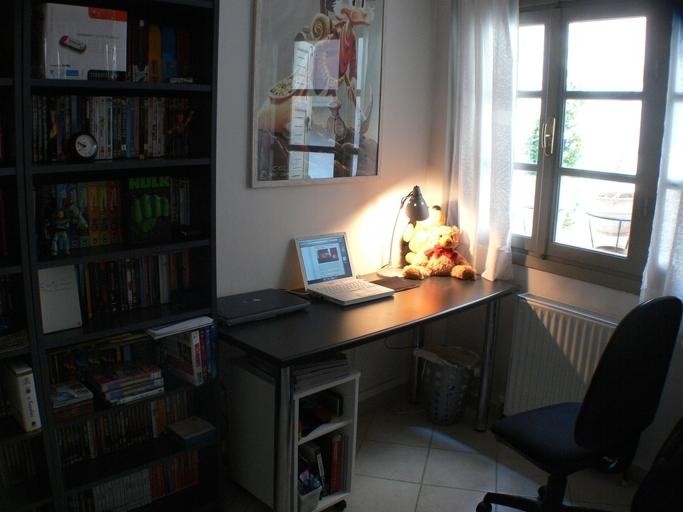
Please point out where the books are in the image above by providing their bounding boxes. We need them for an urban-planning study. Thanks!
[34,23,194,336]
[242,350,350,500]
[1,316,222,511]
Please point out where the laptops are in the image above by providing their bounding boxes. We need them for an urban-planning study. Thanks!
[217,289,310,325]
[293,232,395,305]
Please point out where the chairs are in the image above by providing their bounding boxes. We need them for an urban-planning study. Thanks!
[476,297,683,512]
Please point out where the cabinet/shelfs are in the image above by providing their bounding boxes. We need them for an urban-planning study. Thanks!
[0,76,53,512]
[31,79,217,498]
[226,355,362,512]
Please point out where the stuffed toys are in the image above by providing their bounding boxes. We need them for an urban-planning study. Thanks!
[402,223,475,282]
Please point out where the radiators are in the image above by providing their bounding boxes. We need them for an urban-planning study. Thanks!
[503,292,619,416]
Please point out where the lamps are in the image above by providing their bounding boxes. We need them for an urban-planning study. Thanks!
[376,185,430,277]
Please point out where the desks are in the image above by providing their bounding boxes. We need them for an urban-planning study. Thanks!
[216,269,519,512]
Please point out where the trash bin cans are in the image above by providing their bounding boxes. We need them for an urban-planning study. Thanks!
[421,345,480,425]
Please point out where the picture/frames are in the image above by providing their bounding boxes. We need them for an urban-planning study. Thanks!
[250,1,386,189]
